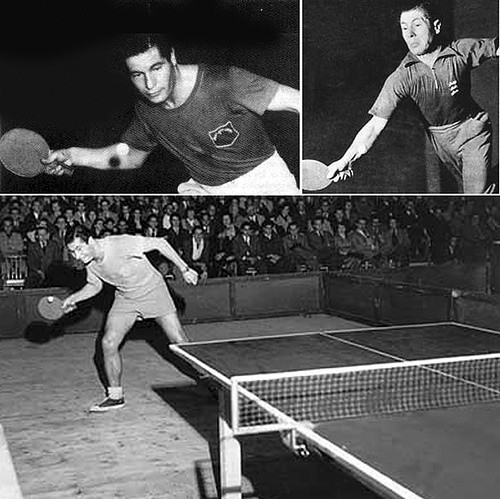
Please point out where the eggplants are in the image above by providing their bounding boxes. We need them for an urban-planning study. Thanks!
[37,296,77,320]
[0,127,74,178]
[302,159,353,191]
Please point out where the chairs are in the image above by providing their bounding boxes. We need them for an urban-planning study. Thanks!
[88,396,127,411]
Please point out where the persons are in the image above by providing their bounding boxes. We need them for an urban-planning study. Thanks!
[0,196,25,215]
[51,214,74,248]
[97,198,120,226]
[22,198,45,233]
[41,32,299,194]
[280,221,318,273]
[371,218,500,267]
[331,221,361,271]
[351,218,376,267]
[257,219,291,275]
[180,206,200,234]
[232,219,269,276]
[165,213,189,256]
[98,229,113,238]
[90,217,106,239]
[181,225,210,279]
[111,226,121,235]
[60,223,222,413]
[116,218,130,235]
[141,213,168,239]
[29,216,61,244]
[25,225,65,288]
[327,0,499,195]
[82,208,98,230]
[198,213,216,241]
[153,260,175,281]
[5,208,26,234]
[0,216,28,283]
[304,215,347,272]
[103,217,114,229]
[207,222,238,278]
[48,200,64,222]
[222,212,239,241]
[118,201,138,236]
[72,200,89,225]
[129,207,146,236]
[61,207,81,227]
